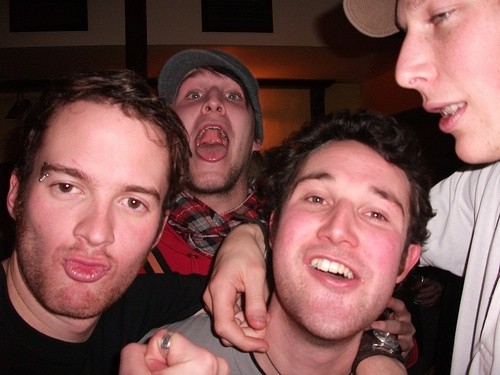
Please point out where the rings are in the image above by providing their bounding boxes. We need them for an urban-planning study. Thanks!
[119,110,435,375]
[162,332,173,353]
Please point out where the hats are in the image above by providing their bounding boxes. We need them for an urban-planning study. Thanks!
[343,0,403,38]
[157,48,264,143]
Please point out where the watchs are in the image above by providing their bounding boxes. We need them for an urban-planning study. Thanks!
[350,328,406,368]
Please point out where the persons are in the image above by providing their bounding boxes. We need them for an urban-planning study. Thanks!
[202,0,500,375]
[0,70,192,375]
[132,49,277,276]
